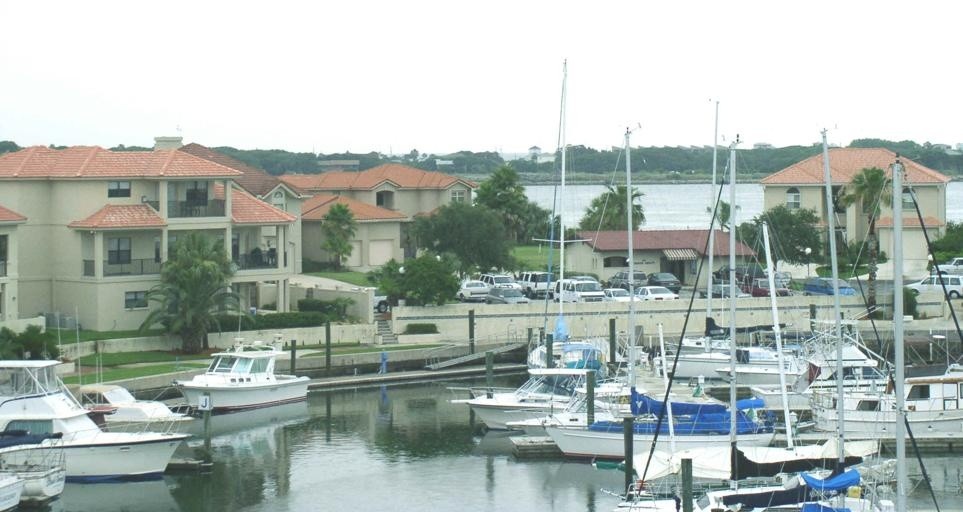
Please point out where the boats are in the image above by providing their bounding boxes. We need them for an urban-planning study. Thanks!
[0,470,24,512]
[167,435,215,472]
[56,478,182,512]
[184,397,311,468]
[169,296,319,412]
[0,358,193,481]
[0,428,68,501]
[52,306,193,435]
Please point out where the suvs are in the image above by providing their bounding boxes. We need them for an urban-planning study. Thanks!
[363,285,389,315]
